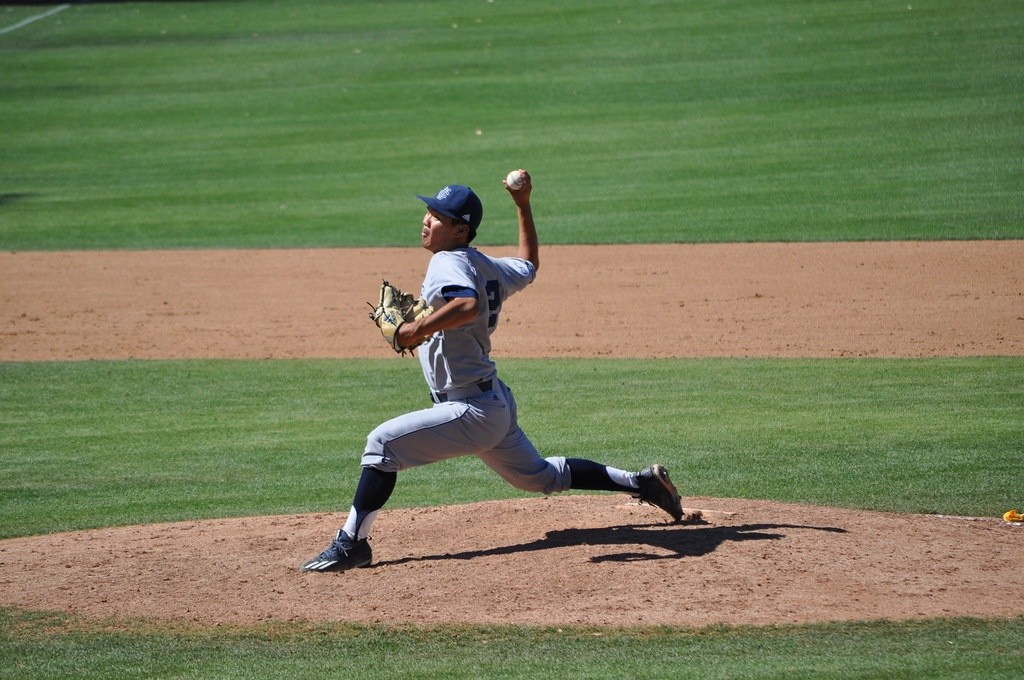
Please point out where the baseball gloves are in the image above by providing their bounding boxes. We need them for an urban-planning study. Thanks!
[368,280,435,354]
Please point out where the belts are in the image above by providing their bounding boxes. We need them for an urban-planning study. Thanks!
[429,380,492,403]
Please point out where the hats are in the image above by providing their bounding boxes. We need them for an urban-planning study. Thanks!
[416,184,483,229]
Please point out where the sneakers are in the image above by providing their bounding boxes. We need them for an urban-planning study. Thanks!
[301,529,373,572]
[632,464,685,520]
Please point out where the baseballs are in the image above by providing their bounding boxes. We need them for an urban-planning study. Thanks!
[506,170,524,190]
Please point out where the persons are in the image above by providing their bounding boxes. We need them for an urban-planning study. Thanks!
[297,169,686,573]
